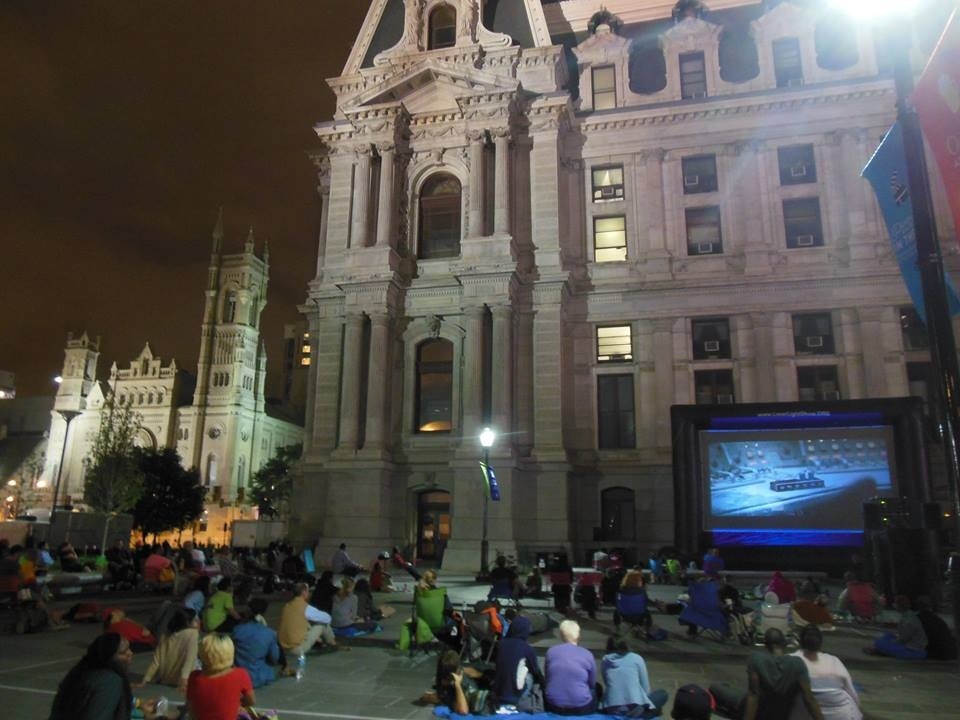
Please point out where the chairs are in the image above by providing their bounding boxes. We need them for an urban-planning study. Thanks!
[688,580,733,641]
[615,591,656,644]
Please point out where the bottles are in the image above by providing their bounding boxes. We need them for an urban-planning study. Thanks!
[296,649,306,681]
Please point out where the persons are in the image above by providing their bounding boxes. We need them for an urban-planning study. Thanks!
[505,609,560,636]
[436,616,668,717]
[482,547,908,641]
[10,569,384,687]
[710,627,824,720]
[0,537,315,593]
[372,544,422,593]
[47,631,133,720]
[785,625,865,720]
[186,631,254,720]
[672,681,725,720]
[415,570,452,616]
[863,598,956,661]
[330,542,368,576]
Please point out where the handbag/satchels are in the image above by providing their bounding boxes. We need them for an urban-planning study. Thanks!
[669,684,716,720]
[709,679,747,720]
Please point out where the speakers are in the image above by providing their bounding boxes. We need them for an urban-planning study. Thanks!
[593,528,608,542]
[923,503,942,528]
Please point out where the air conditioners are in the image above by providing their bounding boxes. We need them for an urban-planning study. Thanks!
[797,234,814,246]
[806,335,823,347]
[704,340,720,352]
[697,241,713,253]
[684,176,699,186]
[791,166,806,177]
[716,393,733,405]
[822,390,840,401]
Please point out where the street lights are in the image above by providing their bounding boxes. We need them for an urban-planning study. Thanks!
[46,408,82,541]
[475,426,500,584]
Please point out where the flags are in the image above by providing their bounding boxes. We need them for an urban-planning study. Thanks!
[860,5,960,362]
